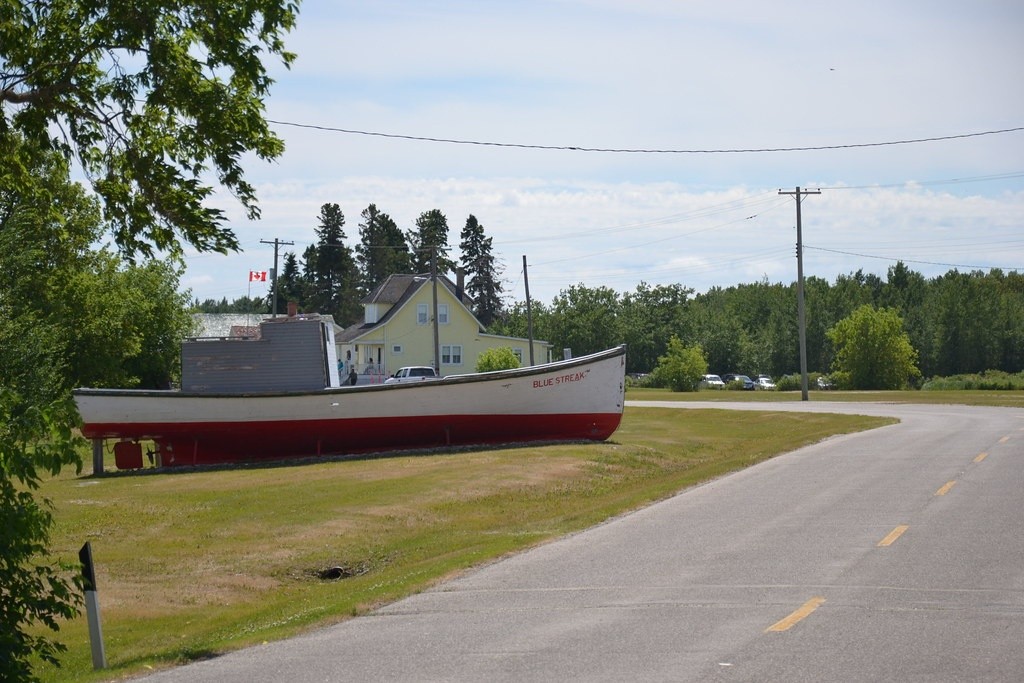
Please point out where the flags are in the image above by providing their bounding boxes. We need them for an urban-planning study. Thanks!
[250,271,266,282]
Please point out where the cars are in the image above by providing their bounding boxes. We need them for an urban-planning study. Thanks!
[700,374,725,390]
[725,376,755,391]
[815,378,837,390]
[753,378,777,392]
[629,374,648,384]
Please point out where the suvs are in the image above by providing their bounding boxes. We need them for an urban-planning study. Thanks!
[383,366,435,385]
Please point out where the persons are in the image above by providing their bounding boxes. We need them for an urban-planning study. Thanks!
[347,369,357,386]
[363,358,373,374]
[338,358,343,378]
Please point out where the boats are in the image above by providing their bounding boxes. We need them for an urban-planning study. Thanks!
[73,343,626,465]
